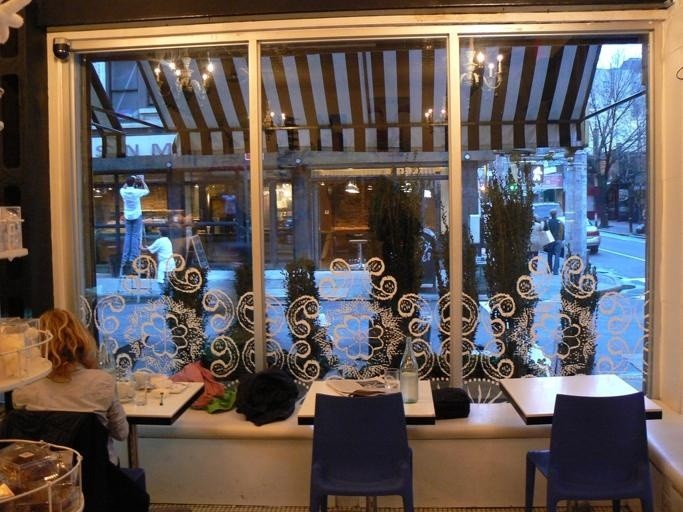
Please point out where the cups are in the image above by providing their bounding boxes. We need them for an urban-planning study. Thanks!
[384,368,399,394]
[136,383,147,405]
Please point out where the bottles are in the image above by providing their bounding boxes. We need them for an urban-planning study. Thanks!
[399,337,419,403]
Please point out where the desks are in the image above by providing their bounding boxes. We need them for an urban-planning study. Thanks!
[495,365,663,440]
[116,374,205,472]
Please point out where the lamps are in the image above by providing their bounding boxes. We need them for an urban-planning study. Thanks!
[150,50,215,105]
[457,36,509,93]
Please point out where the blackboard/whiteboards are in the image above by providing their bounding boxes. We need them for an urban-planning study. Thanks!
[190,234,209,270]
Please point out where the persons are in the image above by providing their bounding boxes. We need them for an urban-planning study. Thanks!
[526,212,544,274]
[220,191,237,234]
[4,305,149,508]
[136,226,175,282]
[542,208,564,276]
[117,174,151,276]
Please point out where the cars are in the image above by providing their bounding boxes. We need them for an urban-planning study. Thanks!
[532,201,600,255]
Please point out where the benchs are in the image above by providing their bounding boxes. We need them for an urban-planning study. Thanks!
[297,371,437,511]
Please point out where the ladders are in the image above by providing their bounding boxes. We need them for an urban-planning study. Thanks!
[115,220,153,304]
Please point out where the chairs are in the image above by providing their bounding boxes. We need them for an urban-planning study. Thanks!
[309,391,415,512]
[521,389,655,511]
[2,405,149,512]
